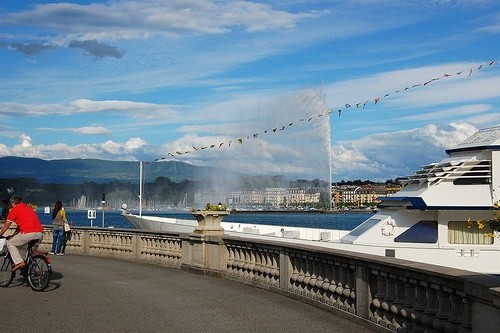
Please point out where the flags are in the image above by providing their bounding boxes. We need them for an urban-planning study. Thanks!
[140,59,499,167]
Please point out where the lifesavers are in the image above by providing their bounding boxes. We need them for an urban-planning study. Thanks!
[381,221,397,236]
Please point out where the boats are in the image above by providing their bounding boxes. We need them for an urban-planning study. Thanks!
[120,124,500,276]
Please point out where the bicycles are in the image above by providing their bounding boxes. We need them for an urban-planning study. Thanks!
[0,235,52,292]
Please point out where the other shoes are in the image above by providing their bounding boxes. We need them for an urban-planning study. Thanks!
[56,253,63,256]
[11,262,25,271]
[48,251,54,254]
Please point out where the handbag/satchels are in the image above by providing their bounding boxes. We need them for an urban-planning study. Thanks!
[64,224,70,231]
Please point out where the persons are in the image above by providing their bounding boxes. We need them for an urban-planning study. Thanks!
[47,201,68,256]
[0,195,43,271]
